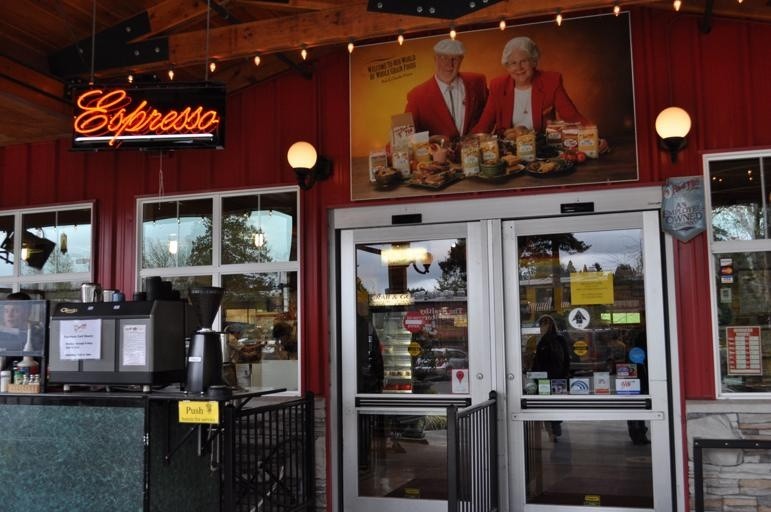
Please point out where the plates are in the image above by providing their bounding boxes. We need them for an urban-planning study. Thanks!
[474,170,511,185]
[524,158,568,178]
[408,170,460,191]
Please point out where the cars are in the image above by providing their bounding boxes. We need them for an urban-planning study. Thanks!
[221,320,269,364]
[414,347,469,381]
[563,327,629,373]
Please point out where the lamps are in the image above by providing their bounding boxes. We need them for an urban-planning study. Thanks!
[288,139,333,190]
[655,107,693,164]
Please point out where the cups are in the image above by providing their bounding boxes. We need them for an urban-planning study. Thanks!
[75,275,180,304]
[1,370,12,393]
[431,147,448,163]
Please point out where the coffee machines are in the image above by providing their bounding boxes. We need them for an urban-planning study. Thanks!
[181,285,243,391]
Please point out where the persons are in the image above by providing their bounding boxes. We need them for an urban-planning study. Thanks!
[0,292,45,356]
[384,38,489,171]
[456,35,612,162]
[224,322,265,362]
[531,311,572,443]
[622,308,653,447]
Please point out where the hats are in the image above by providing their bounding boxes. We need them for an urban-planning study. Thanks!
[433,38,466,57]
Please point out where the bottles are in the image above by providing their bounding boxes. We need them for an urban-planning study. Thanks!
[14,367,41,386]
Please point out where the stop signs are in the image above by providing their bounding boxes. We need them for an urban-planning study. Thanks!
[404,309,426,334]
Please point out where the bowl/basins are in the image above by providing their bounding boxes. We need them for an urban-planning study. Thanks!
[479,158,507,176]
[374,166,401,190]
